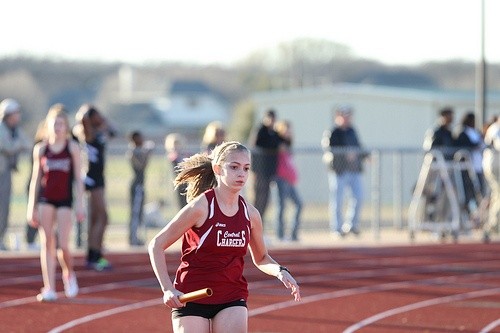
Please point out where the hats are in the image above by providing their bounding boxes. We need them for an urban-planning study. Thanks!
[0,98,21,121]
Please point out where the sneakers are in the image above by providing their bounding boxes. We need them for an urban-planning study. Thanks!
[85,257,113,272]
[63,272,79,298]
[36,288,57,302]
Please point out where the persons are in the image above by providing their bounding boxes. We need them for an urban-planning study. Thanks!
[272,121,303,241]
[251,110,276,235]
[322,106,369,236]
[0,98,226,301]
[149,142,302,333]
[411,108,499,243]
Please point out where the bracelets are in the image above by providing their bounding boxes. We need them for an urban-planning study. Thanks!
[277,267,290,279]
